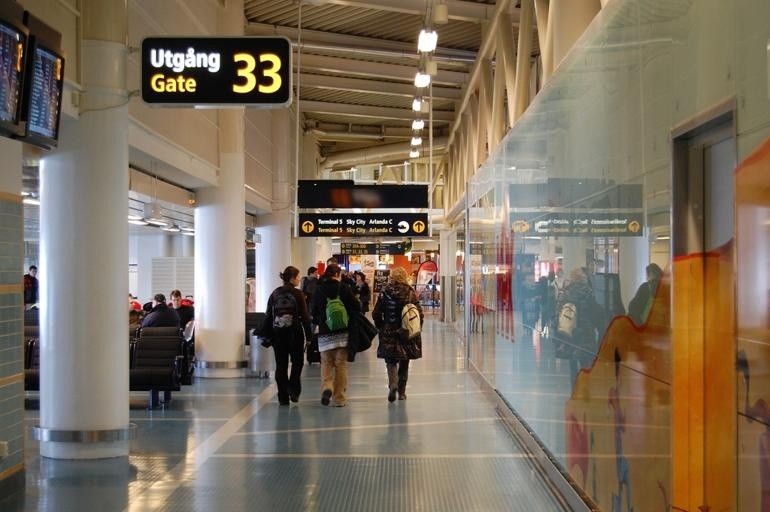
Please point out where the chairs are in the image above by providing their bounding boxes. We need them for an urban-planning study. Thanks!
[127,324,190,412]
[23,322,38,390]
[29,326,43,395]
[126,322,141,367]
[244,310,266,346]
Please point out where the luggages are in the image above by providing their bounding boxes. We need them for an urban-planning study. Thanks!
[306,338,321,364]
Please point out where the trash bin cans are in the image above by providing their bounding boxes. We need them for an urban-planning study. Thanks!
[249,328,274,378]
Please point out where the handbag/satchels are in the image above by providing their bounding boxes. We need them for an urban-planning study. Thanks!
[401,303,421,338]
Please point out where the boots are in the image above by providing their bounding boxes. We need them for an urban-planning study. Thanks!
[389,385,396,403]
[399,387,407,399]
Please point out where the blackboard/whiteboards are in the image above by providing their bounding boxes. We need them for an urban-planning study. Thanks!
[373,270,391,293]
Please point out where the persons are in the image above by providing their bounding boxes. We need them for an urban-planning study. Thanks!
[469,288,486,334]
[258,266,311,406]
[24,265,38,303]
[625,262,662,326]
[129,288,193,340]
[519,265,604,395]
[300,257,371,408]
[372,266,425,402]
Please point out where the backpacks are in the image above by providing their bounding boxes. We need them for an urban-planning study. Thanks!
[272,289,299,329]
[319,283,349,330]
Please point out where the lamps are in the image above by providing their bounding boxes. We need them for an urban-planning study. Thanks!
[399,22,437,161]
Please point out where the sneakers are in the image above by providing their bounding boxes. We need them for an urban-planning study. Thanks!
[334,401,346,407]
[320,388,333,406]
